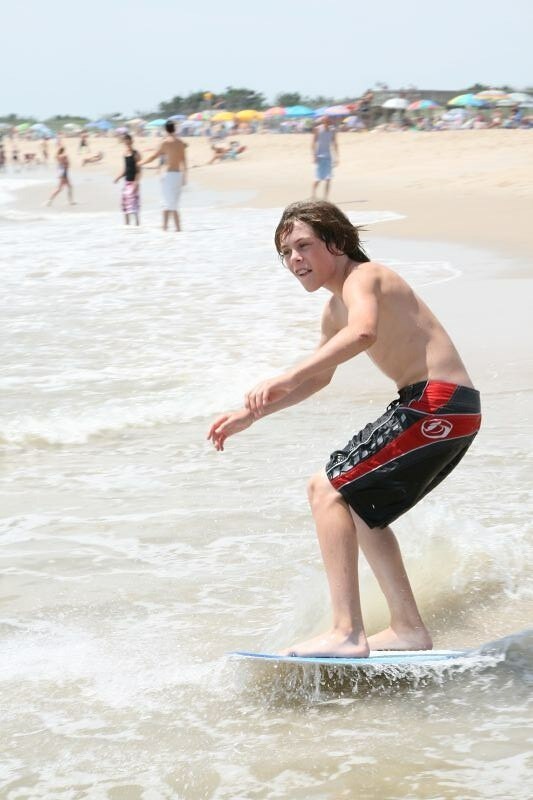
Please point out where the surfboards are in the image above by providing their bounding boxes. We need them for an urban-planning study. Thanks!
[228,629,533,667]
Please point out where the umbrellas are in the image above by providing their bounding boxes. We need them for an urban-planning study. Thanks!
[147,104,350,126]
[0,121,113,132]
[448,89,533,108]
[382,97,440,111]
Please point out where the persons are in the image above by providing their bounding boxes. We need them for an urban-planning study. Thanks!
[310,112,340,200]
[208,144,244,166]
[474,107,521,128]
[136,120,188,232]
[0,130,102,206]
[114,134,140,226]
[206,198,481,658]
